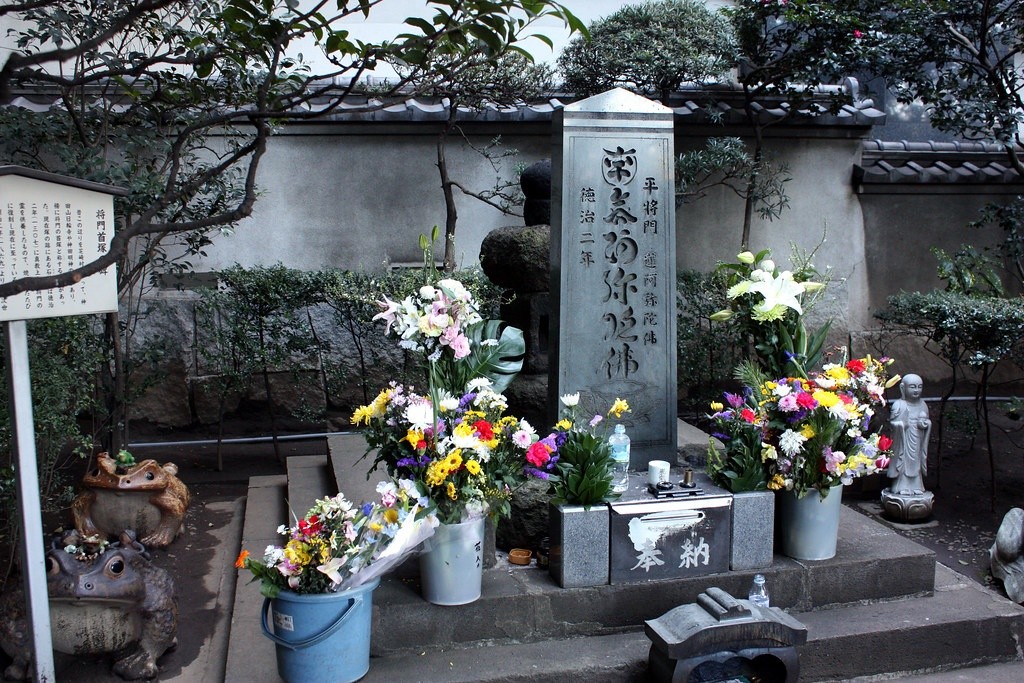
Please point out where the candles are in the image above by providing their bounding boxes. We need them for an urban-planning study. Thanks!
[648,460,670,484]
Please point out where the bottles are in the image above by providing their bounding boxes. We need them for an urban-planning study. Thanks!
[606,424,631,493]
[748,574,770,607]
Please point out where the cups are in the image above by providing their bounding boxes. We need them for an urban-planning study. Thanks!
[648,460,670,486]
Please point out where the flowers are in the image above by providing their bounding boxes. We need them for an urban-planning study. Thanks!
[235,224,633,595]
[703,218,901,502]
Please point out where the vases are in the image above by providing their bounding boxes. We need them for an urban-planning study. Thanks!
[420,518,486,606]
[780,482,843,561]
[483,512,498,570]
[729,488,775,572]
[547,503,609,588]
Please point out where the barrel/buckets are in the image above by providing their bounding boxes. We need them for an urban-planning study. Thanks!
[257,574,381,683]
